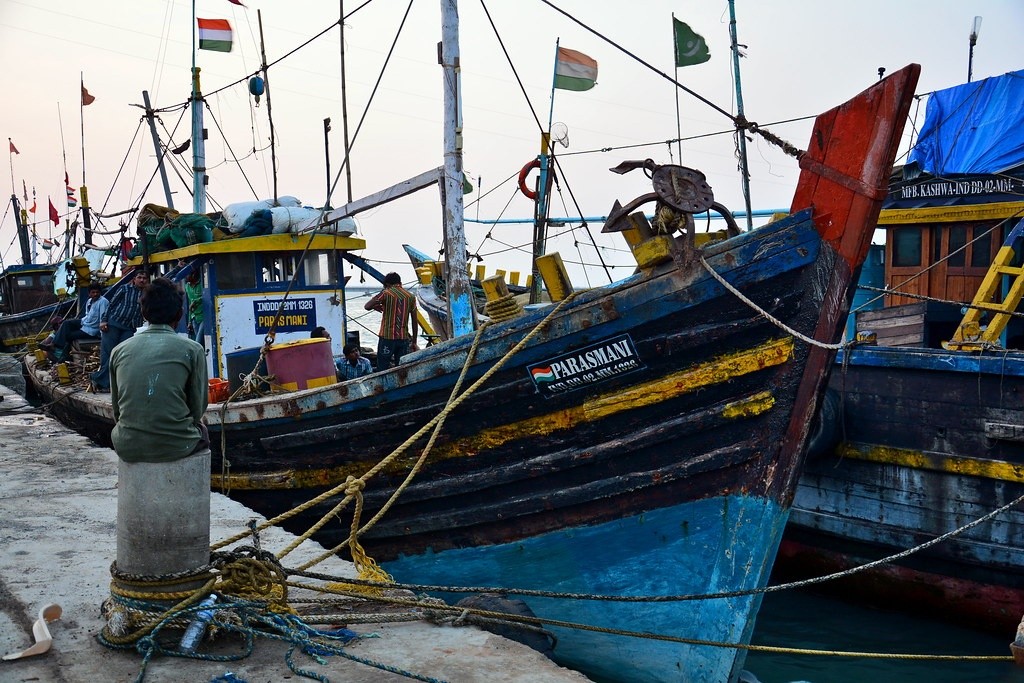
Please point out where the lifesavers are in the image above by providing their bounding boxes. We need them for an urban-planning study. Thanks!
[518,159,552,199]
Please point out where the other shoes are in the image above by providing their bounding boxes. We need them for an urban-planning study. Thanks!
[38,342,53,351]
[90,374,97,394]
[97,383,104,390]
[49,354,57,361]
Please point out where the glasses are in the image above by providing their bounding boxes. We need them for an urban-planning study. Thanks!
[137,276,147,280]
[90,291,99,294]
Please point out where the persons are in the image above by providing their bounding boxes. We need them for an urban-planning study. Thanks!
[37,259,206,396]
[364,271,418,372]
[309,325,374,383]
[108,278,210,463]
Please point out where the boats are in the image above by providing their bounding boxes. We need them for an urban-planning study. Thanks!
[0,0,922,682]
[1,133,125,353]
[400,14,1024,648]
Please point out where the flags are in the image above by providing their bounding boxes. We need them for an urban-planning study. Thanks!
[196,16,232,52]
[672,15,711,68]
[66,186,78,207]
[554,45,598,90]
[42,238,53,250]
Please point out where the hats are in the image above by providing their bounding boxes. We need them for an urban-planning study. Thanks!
[343,343,358,356]
